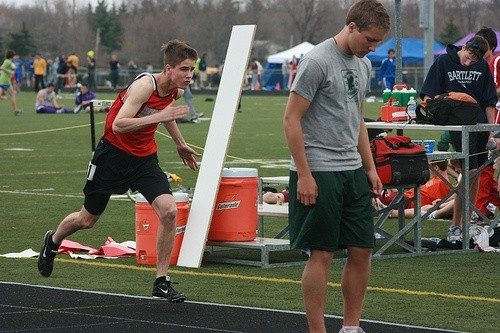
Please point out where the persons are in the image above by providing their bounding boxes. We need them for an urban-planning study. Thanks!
[0,49,23,116]
[86,49,96,88]
[34,83,82,113]
[261,186,290,208]
[11,52,81,94]
[75,83,97,111]
[108,52,120,91]
[142,62,154,74]
[280,0,385,333]
[179,51,308,123]
[126,57,139,86]
[364,24,500,246]
[37,40,203,303]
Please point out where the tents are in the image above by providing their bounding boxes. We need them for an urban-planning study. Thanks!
[362,37,445,95]
[266,41,318,91]
[432,30,500,77]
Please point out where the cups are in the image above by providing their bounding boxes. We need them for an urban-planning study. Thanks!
[423,140,435,153]
[412,140,422,147]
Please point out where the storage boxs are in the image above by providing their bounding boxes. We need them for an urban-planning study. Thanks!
[91,99,102,113]
[411,140,436,153]
[102,100,115,113]
[381,106,408,122]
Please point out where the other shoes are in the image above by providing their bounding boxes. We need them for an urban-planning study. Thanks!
[421,210,441,219]
[372,197,393,218]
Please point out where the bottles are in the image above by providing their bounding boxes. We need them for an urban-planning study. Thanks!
[375,233,385,239]
[382,87,416,106]
[487,138,497,160]
[421,237,442,245]
[407,97,416,122]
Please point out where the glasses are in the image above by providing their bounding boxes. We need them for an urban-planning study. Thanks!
[467,42,487,55]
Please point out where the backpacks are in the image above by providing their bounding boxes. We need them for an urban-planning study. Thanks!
[406,92,482,125]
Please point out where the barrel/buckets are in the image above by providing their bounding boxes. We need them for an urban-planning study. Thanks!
[135,192,189,267]
[208,166,259,242]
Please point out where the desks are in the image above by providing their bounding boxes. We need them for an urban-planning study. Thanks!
[362,120,500,254]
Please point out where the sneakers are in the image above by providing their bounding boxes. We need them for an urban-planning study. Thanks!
[152,275,187,304]
[446,225,463,244]
[38,230,61,277]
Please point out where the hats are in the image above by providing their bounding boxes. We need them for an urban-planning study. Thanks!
[88,51,94,58]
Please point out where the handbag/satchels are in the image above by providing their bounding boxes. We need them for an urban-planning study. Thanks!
[370,135,428,189]
